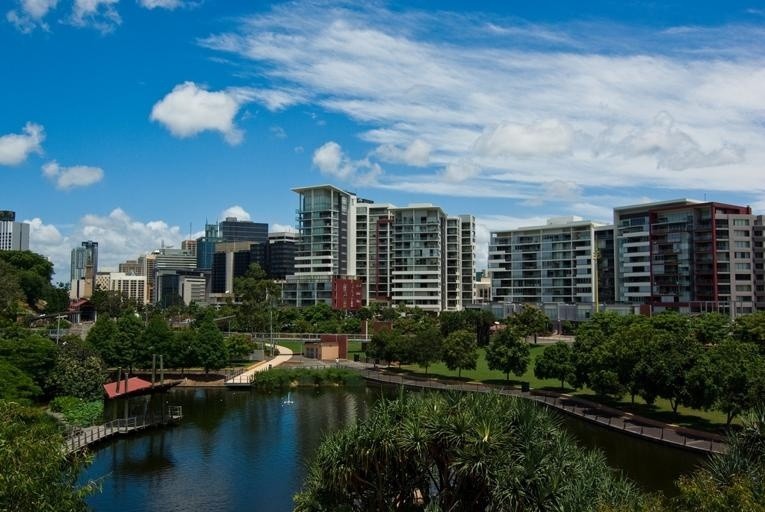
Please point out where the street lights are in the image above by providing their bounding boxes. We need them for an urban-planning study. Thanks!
[56,313,68,345]
